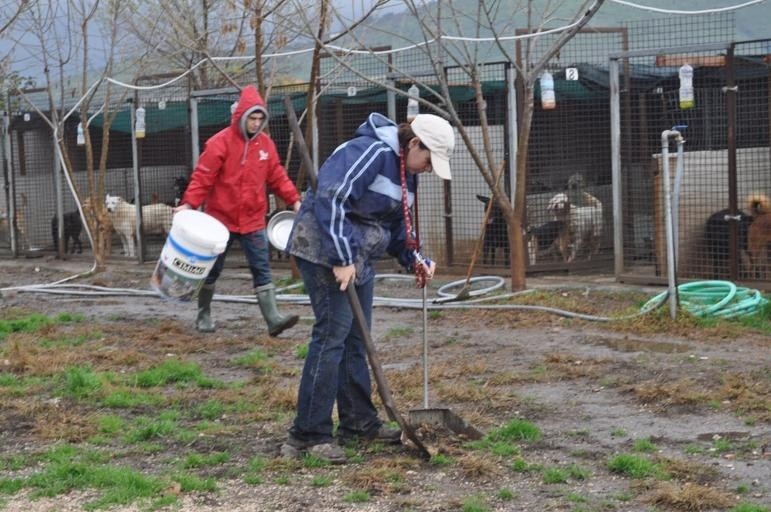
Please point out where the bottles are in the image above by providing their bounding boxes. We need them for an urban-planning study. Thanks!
[136,103,145,139]
[407,84,419,122]
[77,122,85,146]
[679,60,693,110]
[231,102,239,113]
[541,70,555,110]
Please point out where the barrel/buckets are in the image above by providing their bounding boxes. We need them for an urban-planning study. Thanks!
[150,208,229,305]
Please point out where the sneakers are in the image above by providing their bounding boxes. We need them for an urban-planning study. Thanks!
[337,423,402,446]
[281,435,350,463]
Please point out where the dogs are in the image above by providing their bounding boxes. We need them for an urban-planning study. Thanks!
[82,197,120,260]
[558,171,602,189]
[475,193,516,272]
[171,175,190,208]
[104,194,190,258]
[704,193,766,278]
[549,193,605,266]
[49,208,86,257]
[518,220,573,266]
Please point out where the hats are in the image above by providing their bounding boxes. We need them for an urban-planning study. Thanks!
[409,113,456,180]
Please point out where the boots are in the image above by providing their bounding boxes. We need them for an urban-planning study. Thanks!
[252,282,300,340]
[195,283,216,333]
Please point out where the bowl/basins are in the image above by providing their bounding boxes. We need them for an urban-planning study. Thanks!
[266,210,297,252]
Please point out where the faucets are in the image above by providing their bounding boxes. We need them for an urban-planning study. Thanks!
[660,123,690,148]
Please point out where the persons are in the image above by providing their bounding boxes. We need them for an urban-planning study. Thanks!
[170,86,302,341]
[283,113,455,451]
